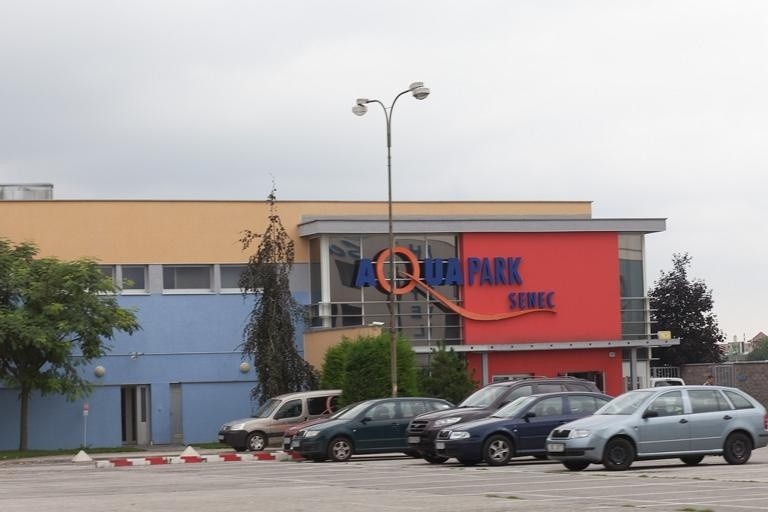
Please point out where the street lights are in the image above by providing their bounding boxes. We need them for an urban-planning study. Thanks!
[352,83,430,397]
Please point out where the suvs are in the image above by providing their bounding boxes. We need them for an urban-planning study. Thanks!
[405,375,601,463]
[218,389,343,451]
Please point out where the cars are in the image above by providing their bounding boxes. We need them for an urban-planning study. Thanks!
[545,386,768,471]
[282,397,455,462]
[434,391,615,465]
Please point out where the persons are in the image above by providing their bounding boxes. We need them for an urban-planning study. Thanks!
[702,376,715,386]
